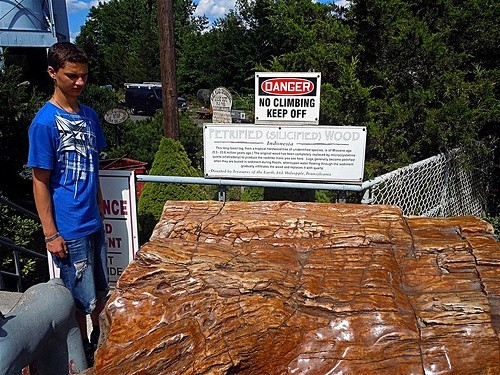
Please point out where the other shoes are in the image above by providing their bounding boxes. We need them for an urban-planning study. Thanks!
[82,342,100,369]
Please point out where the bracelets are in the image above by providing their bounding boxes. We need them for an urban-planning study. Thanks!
[45,232,60,242]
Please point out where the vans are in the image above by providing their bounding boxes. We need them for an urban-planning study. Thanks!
[124,81,186,114]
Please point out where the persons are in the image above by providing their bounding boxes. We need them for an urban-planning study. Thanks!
[27,42,110,351]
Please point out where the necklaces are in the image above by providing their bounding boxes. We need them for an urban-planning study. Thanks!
[52,95,84,132]
